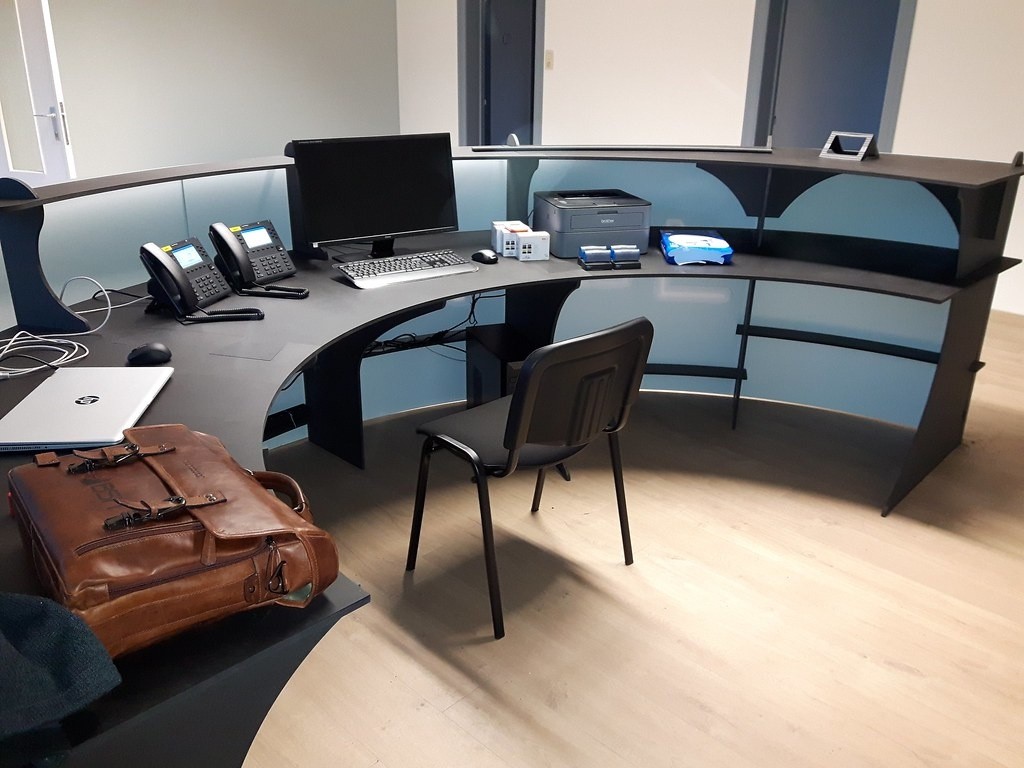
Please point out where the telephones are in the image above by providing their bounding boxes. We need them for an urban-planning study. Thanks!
[139,236,233,317]
[208,217,298,291]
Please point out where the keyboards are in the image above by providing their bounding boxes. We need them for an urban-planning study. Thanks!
[332,249,478,289]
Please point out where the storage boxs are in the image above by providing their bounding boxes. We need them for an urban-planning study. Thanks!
[493,225,530,254]
[501,229,532,257]
[491,220,523,248]
[516,231,550,262]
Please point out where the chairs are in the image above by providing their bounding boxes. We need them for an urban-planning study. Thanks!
[407,317,654,640]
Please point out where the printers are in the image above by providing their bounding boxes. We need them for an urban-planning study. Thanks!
[533,188,653,258]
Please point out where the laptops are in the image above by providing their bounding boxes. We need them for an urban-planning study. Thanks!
[0,367,175,452]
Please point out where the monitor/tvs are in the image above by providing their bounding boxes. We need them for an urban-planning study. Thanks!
[292,132,459,263]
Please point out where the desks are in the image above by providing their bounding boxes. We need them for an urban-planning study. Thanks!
[0,134,1024,768]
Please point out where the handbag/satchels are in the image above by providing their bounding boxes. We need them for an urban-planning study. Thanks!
[7,422,342,661]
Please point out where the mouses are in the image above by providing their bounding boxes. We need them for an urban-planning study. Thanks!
[127,342,171,366]
[472,249,498,264]
